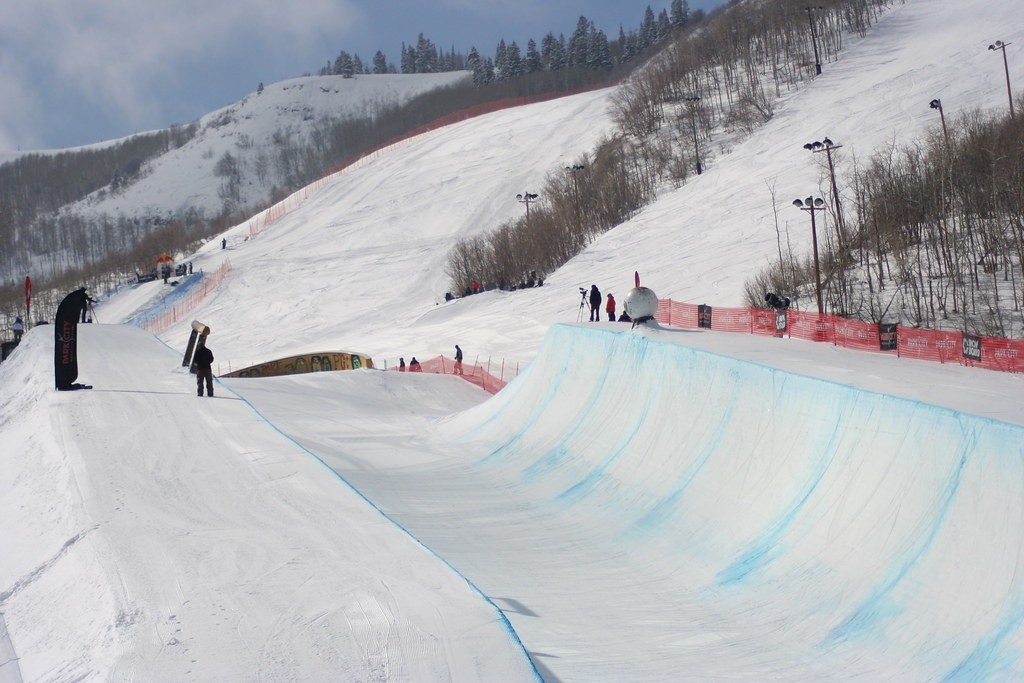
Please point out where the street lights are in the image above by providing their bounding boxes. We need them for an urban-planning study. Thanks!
[565,165,585,224]
[803,137,849,250]
[804,6,822,76]
[685,96,702,175]
[928,99,952,150]
[515,190,539,223]
[792,194,826,320]
[988,40,1015,119]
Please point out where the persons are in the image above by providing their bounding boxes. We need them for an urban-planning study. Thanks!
[618,311,634,322]
[606,293,616,321]
[452,345,462,375]
[589,284,602,322]
[222,238,226,249]
[446,278,544,301]
[188,261,193,274]
[13,317,24,343]
[193,341,214,397]
[151,261,187,284]
[399,358,405,372]
[409,356,422,372]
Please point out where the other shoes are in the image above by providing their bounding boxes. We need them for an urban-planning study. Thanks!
[459,373,463,375]
[453,372,457,374]
[588,320,592,322]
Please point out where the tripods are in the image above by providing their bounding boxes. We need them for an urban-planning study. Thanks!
[576,294,591,323]
